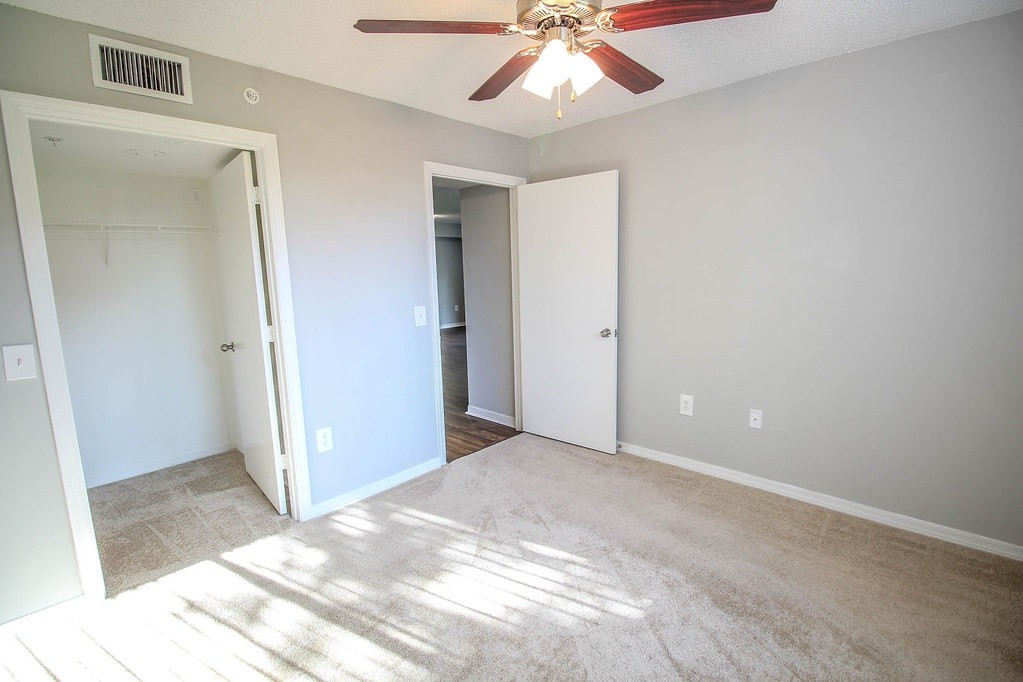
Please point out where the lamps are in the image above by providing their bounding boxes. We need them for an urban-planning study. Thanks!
[515,0,605,119]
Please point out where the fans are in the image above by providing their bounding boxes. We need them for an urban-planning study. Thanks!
[352,0,779,102]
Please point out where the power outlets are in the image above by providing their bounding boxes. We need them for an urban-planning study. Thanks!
[679,394,694,417]
